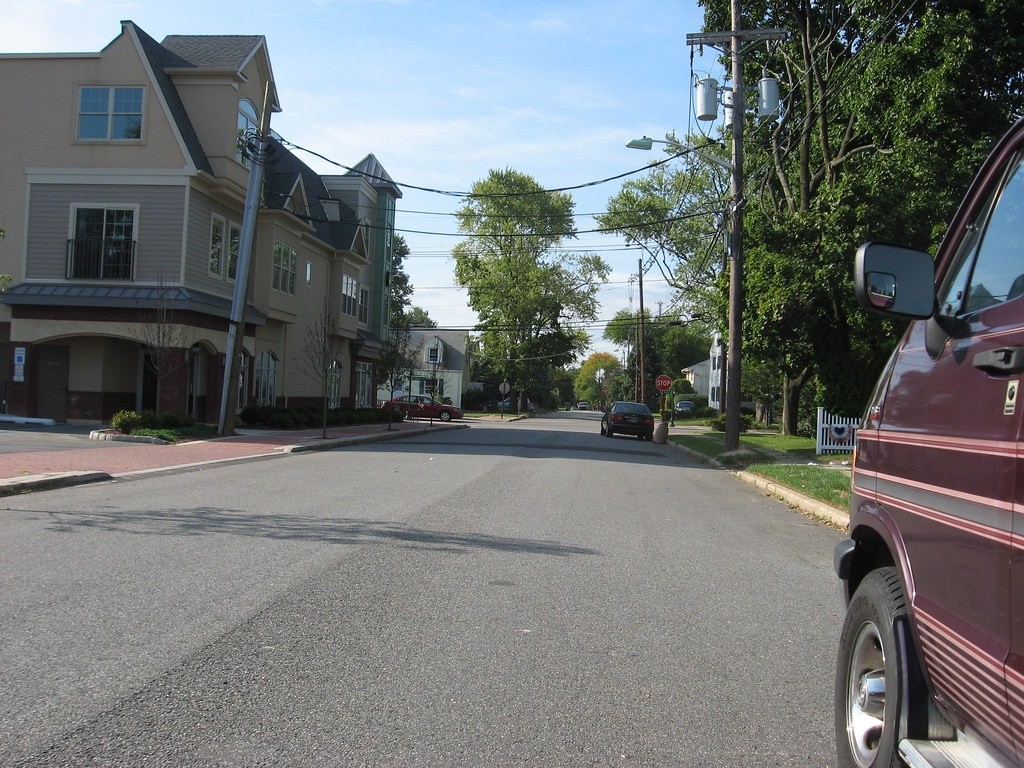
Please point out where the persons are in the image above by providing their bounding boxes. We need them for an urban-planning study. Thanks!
[420,398,425,404]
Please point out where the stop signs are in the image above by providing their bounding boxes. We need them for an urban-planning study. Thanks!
[656,375,672,390]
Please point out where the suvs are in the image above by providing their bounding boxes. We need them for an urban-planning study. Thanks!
[829,113,1023,768]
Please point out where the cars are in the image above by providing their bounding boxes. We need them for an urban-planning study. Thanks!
[381,392,464,422]
[577,402,589,410]
[599,401,655,442]
[674,400,695,419]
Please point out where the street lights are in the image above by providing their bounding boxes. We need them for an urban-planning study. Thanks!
[626,137,748,450]
[614,348,638,404]
